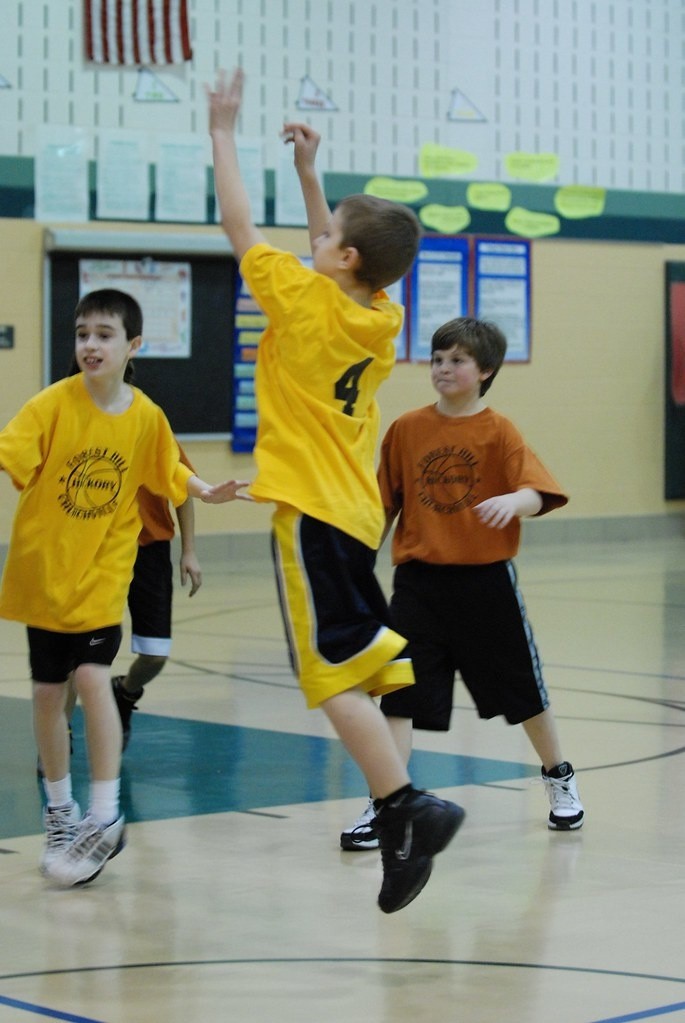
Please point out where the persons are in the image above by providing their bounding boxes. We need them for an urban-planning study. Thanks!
[1,289,243,888]
[204,66,466,913]
[336,314,584,852]
[35,353,202,776]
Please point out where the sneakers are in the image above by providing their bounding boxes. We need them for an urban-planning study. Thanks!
[370,787,465,913]
[111,674,144,754]
[541,761,584,831]
[48,808,126,887]
[37,720,73,780]
[340,797,380,851]
[40,799,82,878]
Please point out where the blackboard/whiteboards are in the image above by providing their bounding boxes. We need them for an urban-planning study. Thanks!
[42,251,236,438]
